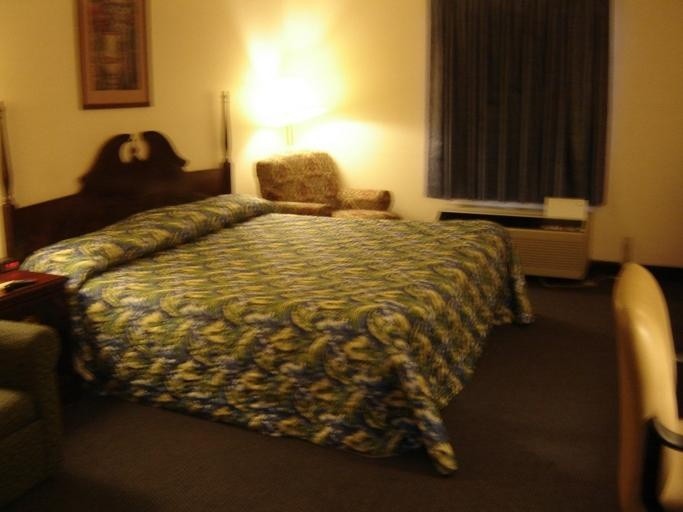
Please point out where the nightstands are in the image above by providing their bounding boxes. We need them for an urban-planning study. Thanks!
[0,269,73,400]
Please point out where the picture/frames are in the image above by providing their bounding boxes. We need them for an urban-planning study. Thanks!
[76,1,153,109]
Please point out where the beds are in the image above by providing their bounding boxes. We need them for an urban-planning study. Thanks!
[1,92,533,473]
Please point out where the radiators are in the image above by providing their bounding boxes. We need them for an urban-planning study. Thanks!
[434,209,588,281]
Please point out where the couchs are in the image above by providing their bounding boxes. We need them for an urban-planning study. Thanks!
[0,320,66,512]
[255,151,399,221]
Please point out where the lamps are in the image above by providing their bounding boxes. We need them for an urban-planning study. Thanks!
[238,56,345,160]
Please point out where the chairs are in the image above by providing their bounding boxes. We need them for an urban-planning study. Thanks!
[611,261,683,512]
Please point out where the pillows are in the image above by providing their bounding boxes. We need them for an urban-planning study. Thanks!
[25,221,182,288]
[152,188,271,236]
